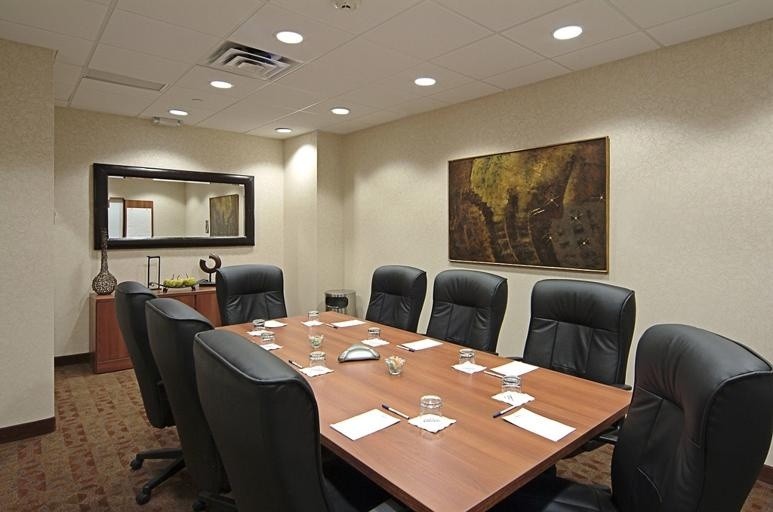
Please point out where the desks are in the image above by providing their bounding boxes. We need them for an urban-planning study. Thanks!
[194,308,637,512]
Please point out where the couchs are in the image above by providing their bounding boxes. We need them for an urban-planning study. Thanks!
[215,266,294,325]
[354,262,428,334]
[489,322,773,512]
[521,280,635,386]
[114,279,209,507]
[422,266,507,357]
[190,333,346,512]
[146,298,233,511]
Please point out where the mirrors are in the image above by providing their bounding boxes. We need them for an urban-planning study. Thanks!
[93,163,255,248]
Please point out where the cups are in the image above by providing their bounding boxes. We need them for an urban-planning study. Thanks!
[309,351,327,375]
[458,349,476,366]
[502,376,522,397]
[384,356,408,375]
[420,395,443,419]
[308,310,320,325]
[251,319,265,332]
[261,331,276,347]
[308,333,325,350]
[368,327,382,342]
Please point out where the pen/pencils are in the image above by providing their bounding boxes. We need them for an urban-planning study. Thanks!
[396,343,414,352]
[289,360,303,369]
[326,322,338,329]
[483,370,501,377]
[381,403,409,419]
[492,405,521,419]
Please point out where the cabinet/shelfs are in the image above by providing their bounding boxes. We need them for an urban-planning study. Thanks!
[87,283,240,374]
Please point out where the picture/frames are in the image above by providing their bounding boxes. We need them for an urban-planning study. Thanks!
[448,133,610,275]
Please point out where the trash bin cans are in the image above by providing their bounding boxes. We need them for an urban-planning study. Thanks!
[325,288,357,320]
